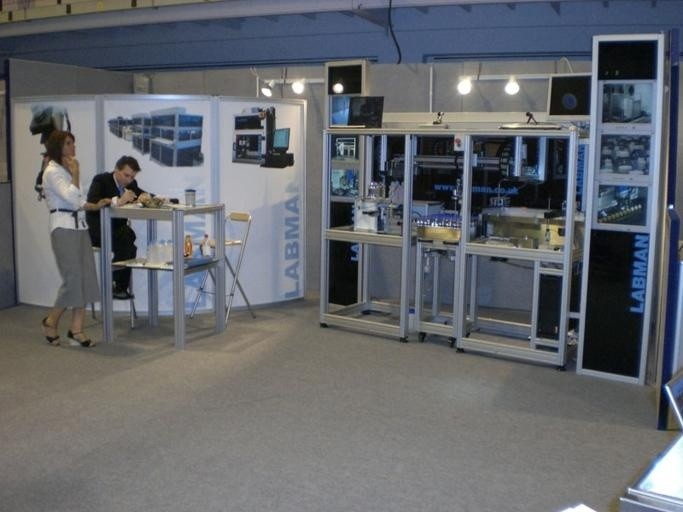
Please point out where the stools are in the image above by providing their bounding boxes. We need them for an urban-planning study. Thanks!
[89,247,138,319]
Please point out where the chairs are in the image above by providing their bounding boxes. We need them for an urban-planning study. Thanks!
[190,213,256,327]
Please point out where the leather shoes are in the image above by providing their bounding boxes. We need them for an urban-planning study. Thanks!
[113,288,132,299]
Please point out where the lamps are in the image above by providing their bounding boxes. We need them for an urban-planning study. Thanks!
[505,76,521,95]
[291,78,306,94]
[259,80,276,97]
[455,77,473,95]
[333,78,345,93]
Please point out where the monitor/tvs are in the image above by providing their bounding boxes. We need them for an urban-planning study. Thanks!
[273,128,290,153]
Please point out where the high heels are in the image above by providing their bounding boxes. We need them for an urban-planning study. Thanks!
[67,330,97,348]
[43,317,59,345]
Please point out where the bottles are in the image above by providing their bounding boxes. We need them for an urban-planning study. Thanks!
[416,213,462,227]
[597,198,643,224]
[148,234,210,263]
[369,181,404,201]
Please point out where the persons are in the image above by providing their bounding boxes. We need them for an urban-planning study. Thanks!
[41,129,112,348]
[85,155,179,300]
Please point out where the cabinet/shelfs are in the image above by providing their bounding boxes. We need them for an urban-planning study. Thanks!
[316,123,588,370]
[99,203,224,350]
